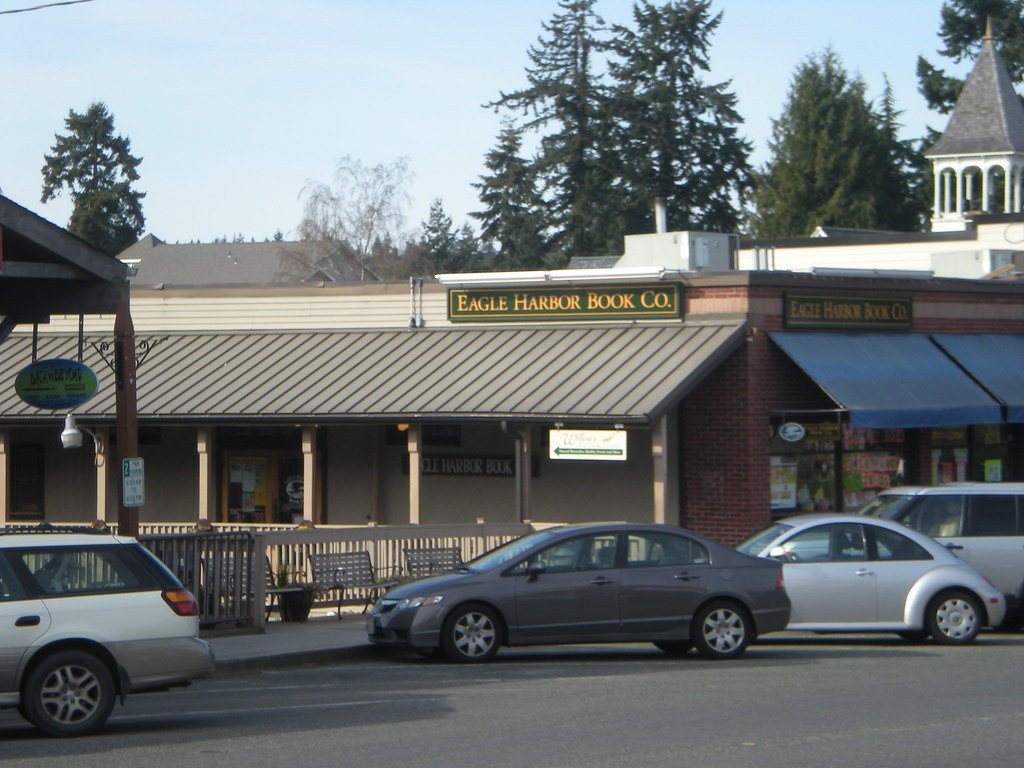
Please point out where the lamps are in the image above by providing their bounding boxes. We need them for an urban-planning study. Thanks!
[61,414,104,467]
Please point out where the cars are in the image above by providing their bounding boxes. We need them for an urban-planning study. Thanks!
[366,524,791,664]
[695,512,1006,645]
[783,480,1024,634]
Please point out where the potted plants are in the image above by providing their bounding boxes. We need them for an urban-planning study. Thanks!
[276,565,315,622]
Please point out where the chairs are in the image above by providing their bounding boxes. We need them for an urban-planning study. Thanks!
[927,514,962,538]
[840,533,864,561]
[598,546,615,569]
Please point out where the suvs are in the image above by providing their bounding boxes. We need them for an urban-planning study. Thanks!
[0,523,215,739]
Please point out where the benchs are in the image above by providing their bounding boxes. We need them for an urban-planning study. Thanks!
[200,554,307,623]
[403,548,466,580]
[305,550,403,619]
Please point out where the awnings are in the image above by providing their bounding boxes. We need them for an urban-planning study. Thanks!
[767,331,1024,428]
[0,319,746,423]
